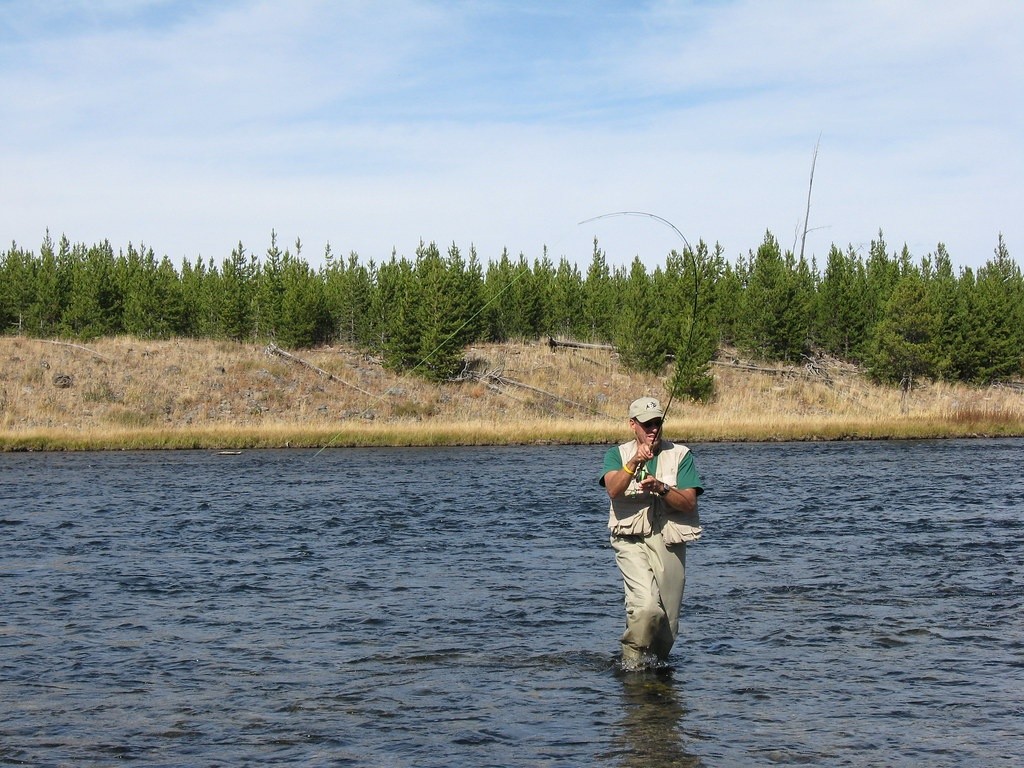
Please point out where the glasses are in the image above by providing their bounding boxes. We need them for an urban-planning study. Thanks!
[633,417,662,428]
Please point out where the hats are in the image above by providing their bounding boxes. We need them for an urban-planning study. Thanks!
[629,397,663,423]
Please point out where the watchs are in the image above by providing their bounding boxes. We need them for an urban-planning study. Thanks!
[659,483,669,496]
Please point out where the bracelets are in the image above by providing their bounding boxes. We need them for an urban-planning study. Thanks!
[624,465,634,475]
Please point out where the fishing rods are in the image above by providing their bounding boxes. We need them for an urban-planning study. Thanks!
[579,211,700,486]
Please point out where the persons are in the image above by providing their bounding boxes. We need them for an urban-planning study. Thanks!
[599,397,704,661]
